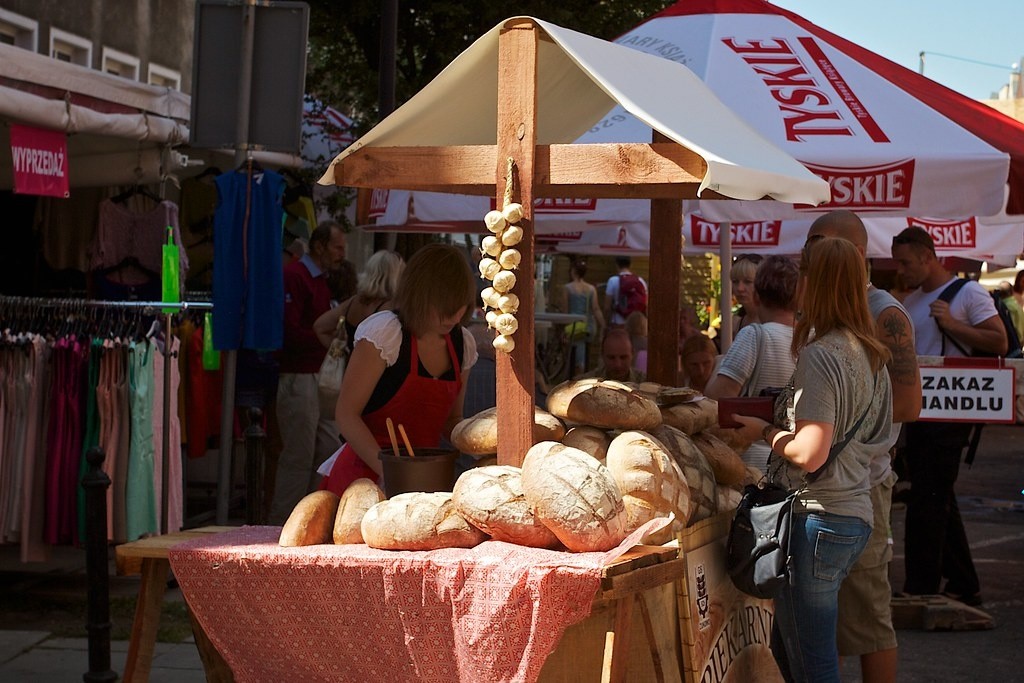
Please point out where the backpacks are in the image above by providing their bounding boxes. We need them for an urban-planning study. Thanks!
[610,274,647,320]
[935,279,1023,465]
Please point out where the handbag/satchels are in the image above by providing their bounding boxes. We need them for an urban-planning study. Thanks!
[584,314,596,342]
[725,481,805,600]
[565,321,588,340]
[318,294,357,400]
[759,386,784,405]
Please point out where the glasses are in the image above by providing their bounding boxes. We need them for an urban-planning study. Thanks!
[804,235,825,248]
[893,236,935,255]
[732,254,763,267]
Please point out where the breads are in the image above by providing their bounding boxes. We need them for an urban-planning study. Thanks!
[278,377,765,552]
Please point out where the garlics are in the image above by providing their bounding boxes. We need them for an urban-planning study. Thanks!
[478,202,524,352]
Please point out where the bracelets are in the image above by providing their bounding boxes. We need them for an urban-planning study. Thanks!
[762,424,780,442]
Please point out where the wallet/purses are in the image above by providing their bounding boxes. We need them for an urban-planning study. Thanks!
[718,396,773,428]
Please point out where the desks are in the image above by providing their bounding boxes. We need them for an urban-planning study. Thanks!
[534,312,586,384]
[116,524,685,683]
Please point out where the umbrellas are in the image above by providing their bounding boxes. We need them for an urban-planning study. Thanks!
[301,0,1024,267]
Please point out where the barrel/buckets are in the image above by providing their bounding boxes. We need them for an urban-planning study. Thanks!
[377,444,460,496]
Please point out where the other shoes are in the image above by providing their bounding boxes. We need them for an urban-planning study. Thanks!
[892,591,910,597]
[939,591,983,606]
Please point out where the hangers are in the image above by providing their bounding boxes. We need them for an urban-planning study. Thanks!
[108,183,162,205]
[186,235,214,249]
[191,263,214,277]
[236,159,261,174]
[194,166,222,180]
[0,292,182,358]
[277,192,312,257]
[101,247,158,276]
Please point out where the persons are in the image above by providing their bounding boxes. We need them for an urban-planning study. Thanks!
[261,220,491,527]
[801,210,923,683]
[703,254,815,475]
[560,253,764,394]
[892,226,1009,607]
[1001,270,1024,423]
[730,236,894,683]
